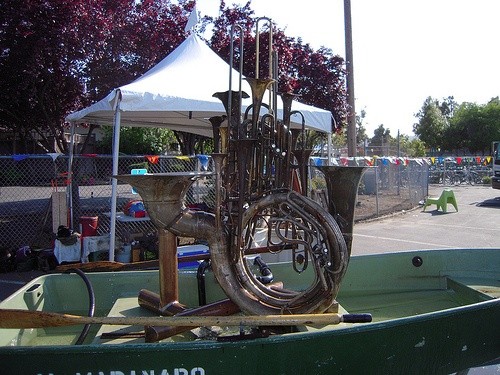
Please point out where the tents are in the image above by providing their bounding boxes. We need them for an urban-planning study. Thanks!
[61,33,338,228]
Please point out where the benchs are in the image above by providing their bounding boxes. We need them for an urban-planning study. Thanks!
[91,297,162,345]
[446,277,500,304]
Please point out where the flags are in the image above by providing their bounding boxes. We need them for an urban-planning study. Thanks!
[185,6,198,34]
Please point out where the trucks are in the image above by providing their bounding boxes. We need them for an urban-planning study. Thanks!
[492,140,500,189]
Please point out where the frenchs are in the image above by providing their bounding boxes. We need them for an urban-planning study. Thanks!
[111,171,349,317]
[232,165,379,308]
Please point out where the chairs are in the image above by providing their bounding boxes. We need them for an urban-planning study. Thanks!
[422,189,458,213]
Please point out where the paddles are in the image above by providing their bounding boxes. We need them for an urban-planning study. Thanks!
[55,243,298,274]
[0,309,375,329]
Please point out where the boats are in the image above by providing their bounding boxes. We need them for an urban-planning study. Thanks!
[0,244,499,375]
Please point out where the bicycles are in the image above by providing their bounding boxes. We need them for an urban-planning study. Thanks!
[421,160,493,187]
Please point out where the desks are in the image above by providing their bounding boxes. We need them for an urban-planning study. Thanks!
[101,211,152,246]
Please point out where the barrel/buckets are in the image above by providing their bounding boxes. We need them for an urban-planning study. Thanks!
[114,242,132,264]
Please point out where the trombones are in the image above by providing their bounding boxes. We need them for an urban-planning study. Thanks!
[213,17,317,273]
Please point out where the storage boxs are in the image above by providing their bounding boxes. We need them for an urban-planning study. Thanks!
[177,245,210,269]
